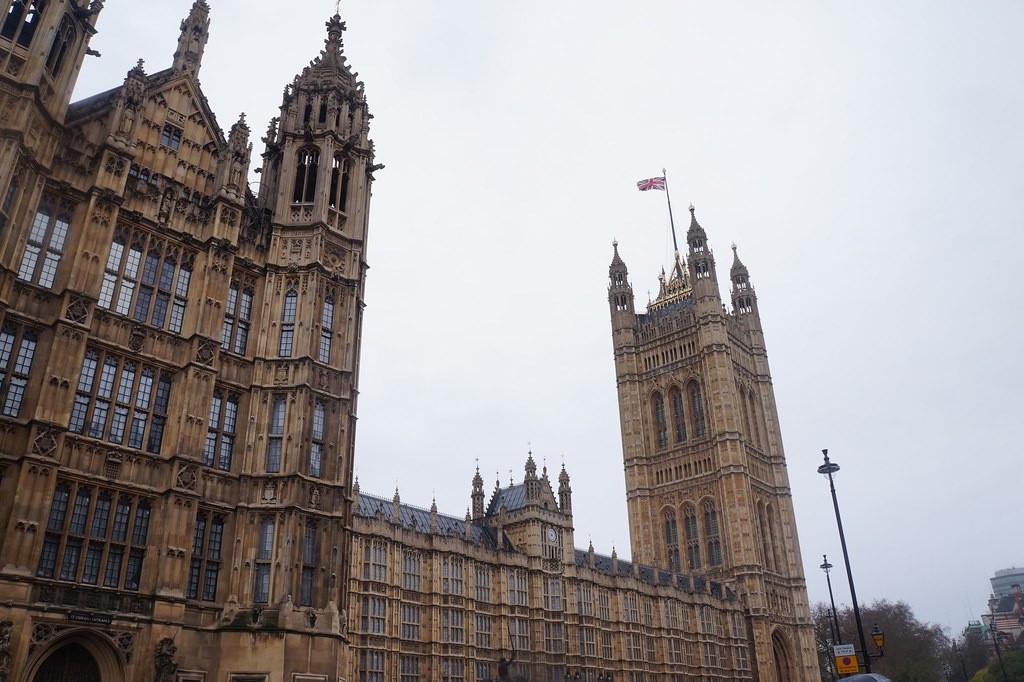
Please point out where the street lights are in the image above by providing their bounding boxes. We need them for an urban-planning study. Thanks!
[942,663,950,682]
[819,554,842,647]
[817,448,885,673]
[957,647,969,682]
[825,609,837,647]
[989,625,1009,682]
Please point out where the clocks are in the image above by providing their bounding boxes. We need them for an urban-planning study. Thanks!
[548,529,557,542]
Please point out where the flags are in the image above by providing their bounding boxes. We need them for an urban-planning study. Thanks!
[637,176,666,191]
